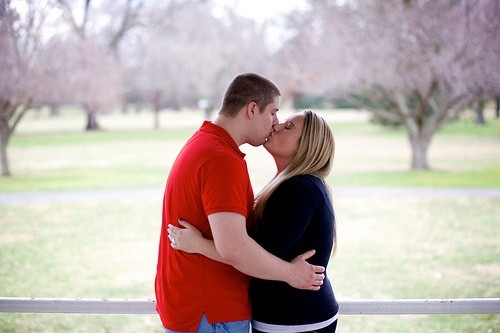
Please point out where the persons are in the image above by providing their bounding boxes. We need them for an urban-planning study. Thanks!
[166,109,340,333]
[153,72,325,332]
